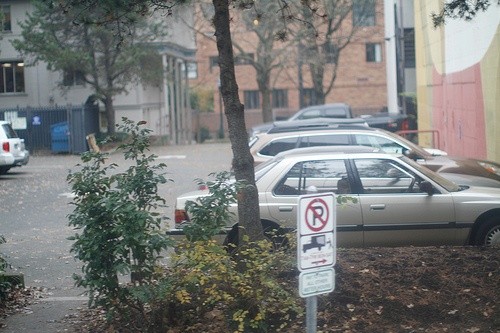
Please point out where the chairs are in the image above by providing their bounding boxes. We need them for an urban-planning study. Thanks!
[337,178,351,194]
[275,185,295,194]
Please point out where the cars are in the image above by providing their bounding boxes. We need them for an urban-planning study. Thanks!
[0,120,30,174]
[198,102,500,189]
[165,149,500,259]
